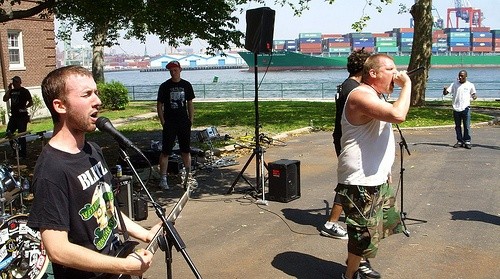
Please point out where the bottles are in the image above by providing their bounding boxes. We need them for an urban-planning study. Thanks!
[23,176,29,198]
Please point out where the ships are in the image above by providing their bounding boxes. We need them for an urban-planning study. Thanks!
[236,48,500,73]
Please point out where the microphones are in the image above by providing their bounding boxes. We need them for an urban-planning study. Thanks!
[406,65,426,77]
[95,116,133,147]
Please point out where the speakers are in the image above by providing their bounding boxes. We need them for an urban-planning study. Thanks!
[113,174,134,221]
[267,158,301,203]
[244,7,277,54]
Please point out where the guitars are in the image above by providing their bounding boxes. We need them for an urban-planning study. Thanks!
[88,166,199,279]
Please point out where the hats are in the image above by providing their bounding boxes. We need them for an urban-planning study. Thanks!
[166,61,181,69]
[11,76,21,82]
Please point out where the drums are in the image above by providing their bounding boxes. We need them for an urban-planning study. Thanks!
[0,160,29,209]
[0,213,50,279]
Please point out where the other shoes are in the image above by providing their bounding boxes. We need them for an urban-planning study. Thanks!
[453,144,462,147]
[465,144,471,148]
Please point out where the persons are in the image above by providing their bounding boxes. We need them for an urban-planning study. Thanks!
[156,61,195,190]
[319,49,371,240]
[3,76,32,159]
[336,54,412,279]
[443,70,477,149]
[27,65,163,279]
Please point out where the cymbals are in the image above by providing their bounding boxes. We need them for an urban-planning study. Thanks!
[0,129,33,145]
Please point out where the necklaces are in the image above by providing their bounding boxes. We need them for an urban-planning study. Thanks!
[363,82,380,94]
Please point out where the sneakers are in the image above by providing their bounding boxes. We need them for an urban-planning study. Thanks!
[159,179,169,189]
[341,270,366,279]
[321,223,348,240]
[346,258,380,279]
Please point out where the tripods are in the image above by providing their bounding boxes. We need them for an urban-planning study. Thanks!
[381,93,428,237]
[225,54,271,194]
